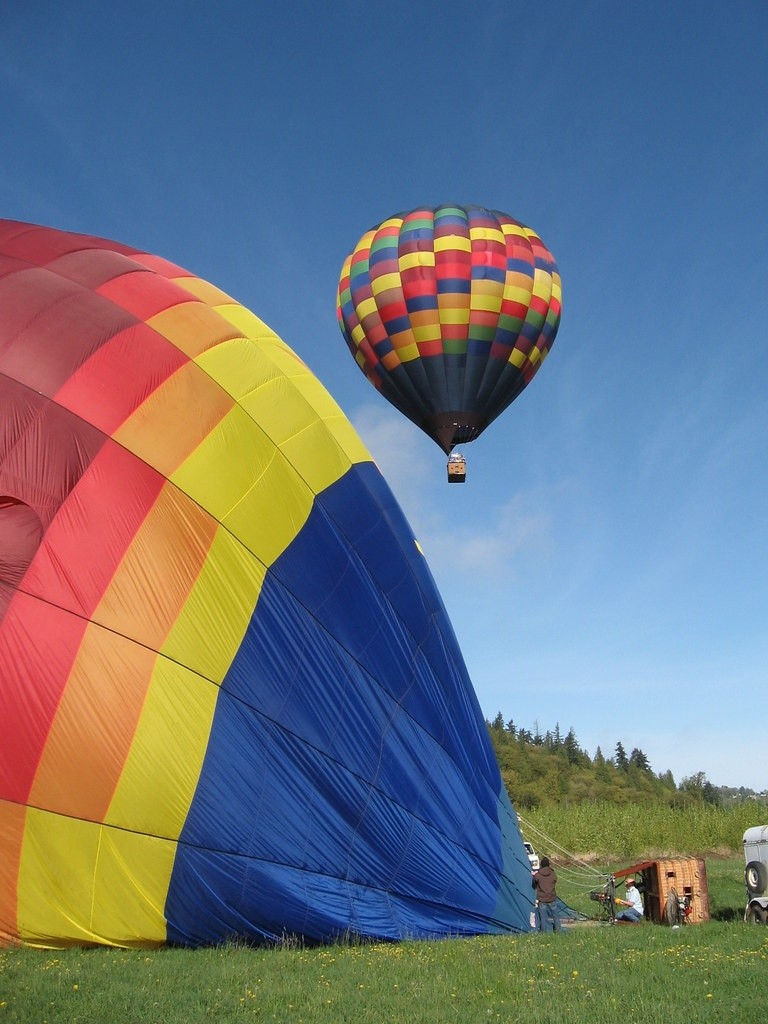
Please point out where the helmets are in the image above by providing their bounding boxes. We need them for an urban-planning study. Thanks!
[625,878,635,885]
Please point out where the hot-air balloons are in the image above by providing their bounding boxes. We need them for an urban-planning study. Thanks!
[0,218,710,950]
[336,203,563,484]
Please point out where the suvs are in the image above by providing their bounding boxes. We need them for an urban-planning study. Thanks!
[524,842,539,871]
[742,825,768,926]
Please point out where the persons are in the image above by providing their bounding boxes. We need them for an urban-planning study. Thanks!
[614,878,643,923]
[532,857,561,934]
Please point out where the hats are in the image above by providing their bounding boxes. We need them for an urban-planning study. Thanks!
[541,859,549,866]
